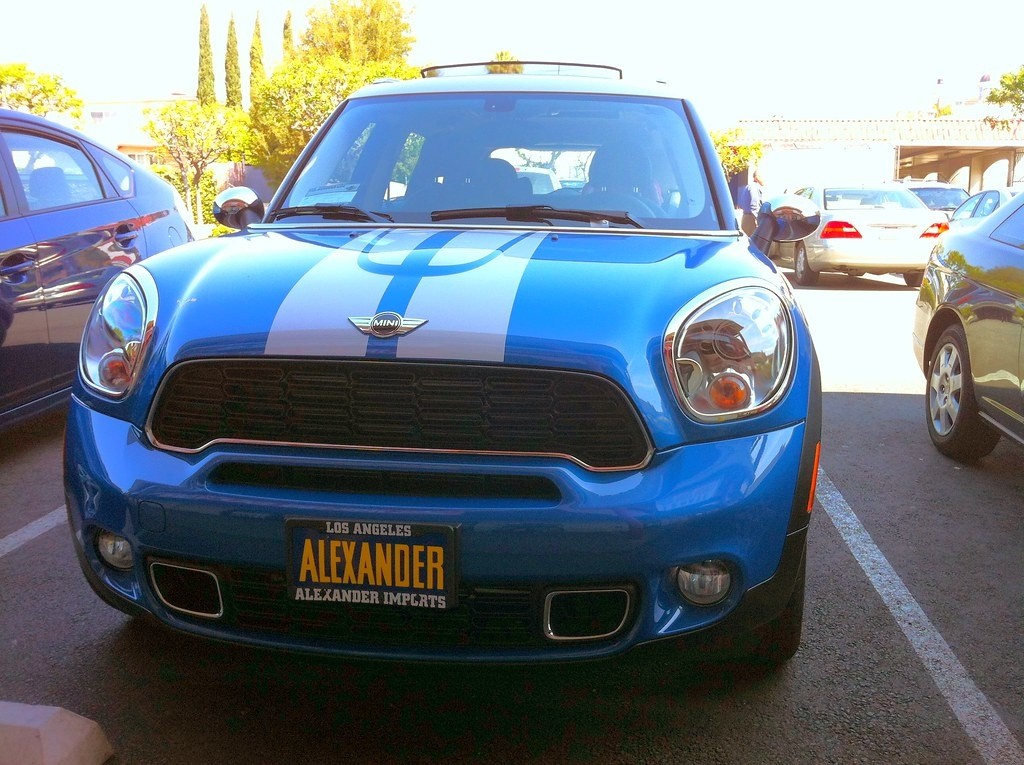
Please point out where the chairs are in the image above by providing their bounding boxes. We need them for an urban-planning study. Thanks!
[28,166,71,210]
[585,141,670,217]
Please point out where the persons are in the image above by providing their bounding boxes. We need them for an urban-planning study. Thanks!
[738,168,764,237]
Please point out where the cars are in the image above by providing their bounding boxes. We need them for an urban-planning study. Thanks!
[892,177,972,222]
[909,190,1024,460]
[0,107,196,433]
[58,73,828,675]
[767,184,949,288]
[953,187,1023,218]
